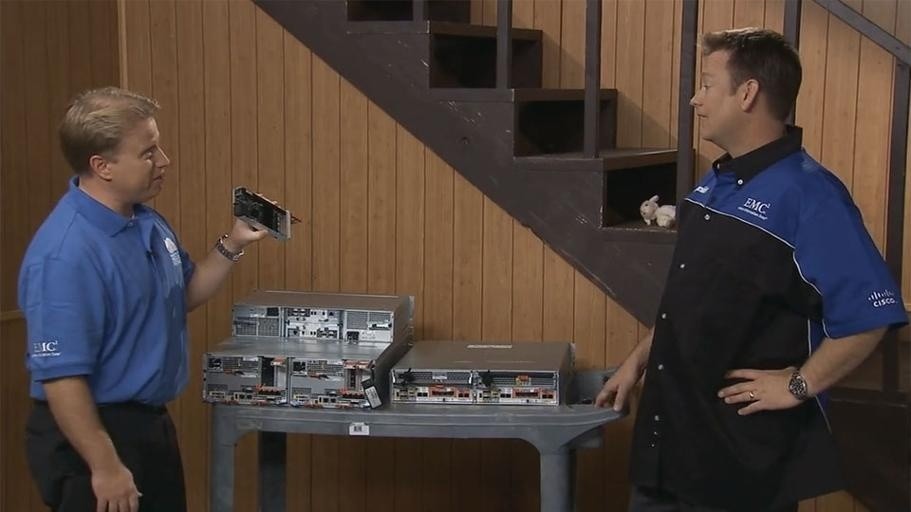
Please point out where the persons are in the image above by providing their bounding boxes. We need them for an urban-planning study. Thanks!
[15,84,283,511]
[593,24,910,510]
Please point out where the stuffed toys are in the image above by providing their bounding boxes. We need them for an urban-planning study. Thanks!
[641,195,677,229]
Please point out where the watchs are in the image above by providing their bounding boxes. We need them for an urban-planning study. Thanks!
[787,365,815,406]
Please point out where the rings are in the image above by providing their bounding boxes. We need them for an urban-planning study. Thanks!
[746,390,755,402]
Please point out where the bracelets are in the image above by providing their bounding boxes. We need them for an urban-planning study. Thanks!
[214,233,246,263]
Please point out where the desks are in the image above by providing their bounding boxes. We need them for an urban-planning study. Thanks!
[207,370,626,512]
